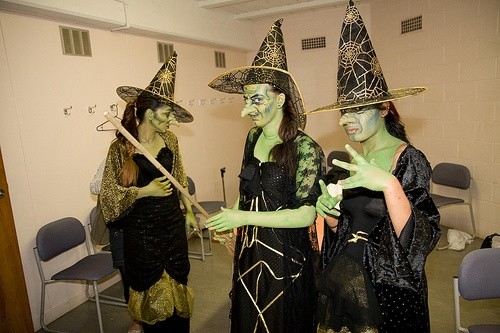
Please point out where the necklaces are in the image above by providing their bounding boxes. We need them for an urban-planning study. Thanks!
[262,132,278,140]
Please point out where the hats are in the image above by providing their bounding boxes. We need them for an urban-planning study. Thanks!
[208,18,307,131]
[303,0,428,115]
[116,51,194,124]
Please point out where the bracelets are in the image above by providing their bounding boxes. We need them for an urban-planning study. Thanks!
[329,225,337,229]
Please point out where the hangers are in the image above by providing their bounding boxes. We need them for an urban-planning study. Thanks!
[96,104,122,131]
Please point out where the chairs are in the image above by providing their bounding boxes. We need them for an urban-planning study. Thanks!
[89,207,127,302]
[453,247,500,333]
[327,151,350,184]
[429,163,476,251]
[32,218,128,333]
[187,217,206,262]
[180,177,226,256]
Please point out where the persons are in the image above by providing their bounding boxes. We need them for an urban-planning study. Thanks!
[314,0,443,333]
[89,157,106,194]
[99,50,200,333]
[203,17,326,333]
